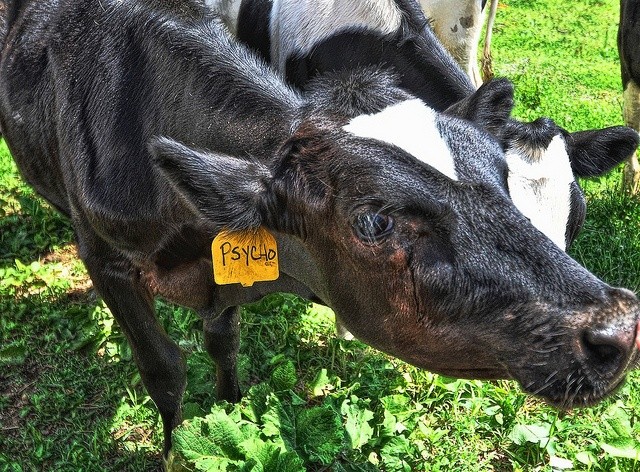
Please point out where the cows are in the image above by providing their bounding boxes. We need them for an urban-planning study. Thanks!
[198,0,640,340]
[415,0,499,92]
[0,0,640,472]
[616,0,640,199]
